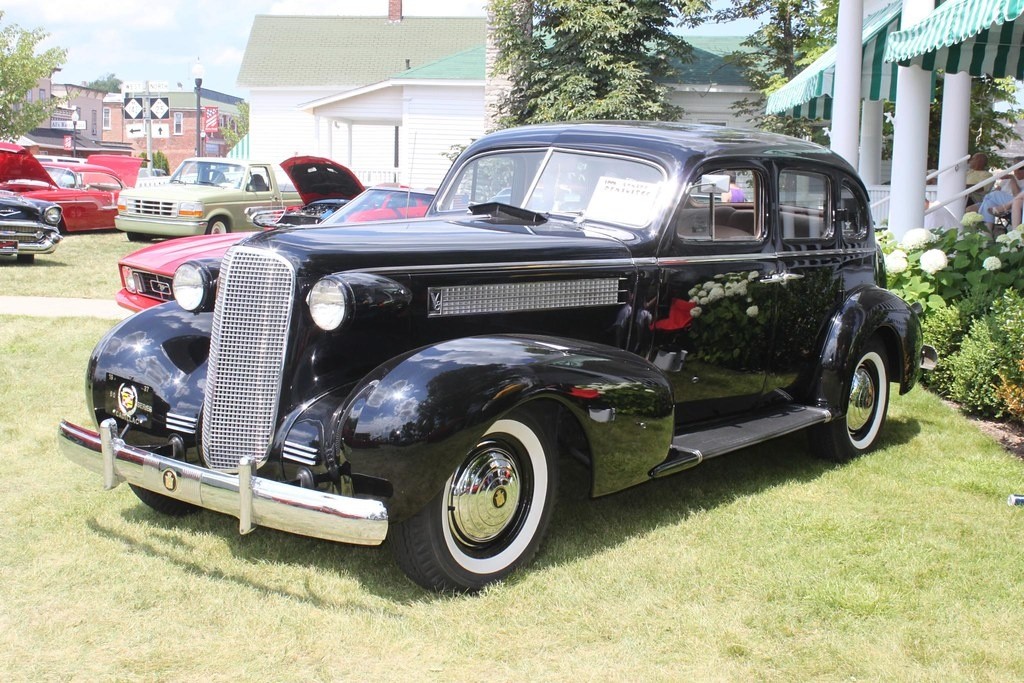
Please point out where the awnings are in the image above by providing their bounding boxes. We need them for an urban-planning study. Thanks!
[884,0,1024,82]
[765,0,939,121]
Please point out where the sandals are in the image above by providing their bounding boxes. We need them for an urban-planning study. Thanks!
[988,204,1010,217]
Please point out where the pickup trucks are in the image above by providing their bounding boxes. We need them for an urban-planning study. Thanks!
[114,155,304,238]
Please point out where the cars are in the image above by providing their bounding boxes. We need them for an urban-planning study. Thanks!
[246,153,440,230]
[57,120,925,593]
[0,190,63,262]
[0,141,151,234]
[137,168,166,178]
[117,180,435,316]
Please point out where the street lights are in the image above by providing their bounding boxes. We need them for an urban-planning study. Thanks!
[192,56,204,157]
[71,111,80,156]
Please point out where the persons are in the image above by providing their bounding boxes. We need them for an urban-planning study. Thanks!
[721,171,748,202]
[615,270,663,353]
[832,305,858,374]
[924,152,1024,232]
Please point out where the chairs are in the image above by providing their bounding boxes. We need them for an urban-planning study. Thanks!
[732,210,823,239]
[247,173,267,192]
[679,205,733,227]
[968,181,1011,234]
